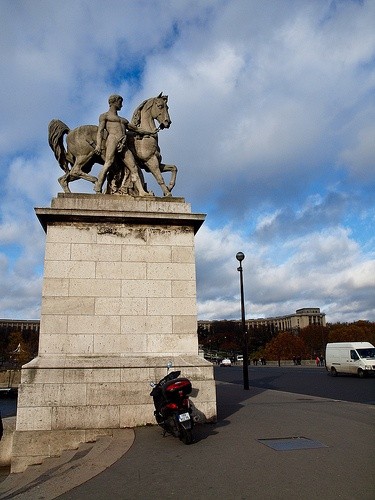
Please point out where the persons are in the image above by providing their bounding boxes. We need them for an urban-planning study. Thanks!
[212,355,301,367]
[94,95,153,196]
[316,355,323,367]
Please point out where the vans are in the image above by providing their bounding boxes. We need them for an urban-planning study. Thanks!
[325,342,375,376]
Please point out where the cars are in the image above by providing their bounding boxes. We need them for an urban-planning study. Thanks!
[198,348,243,367]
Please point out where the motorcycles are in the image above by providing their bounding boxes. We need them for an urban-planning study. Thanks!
[150,371,202,445]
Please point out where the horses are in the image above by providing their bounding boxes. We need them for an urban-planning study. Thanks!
[47,91,178,196]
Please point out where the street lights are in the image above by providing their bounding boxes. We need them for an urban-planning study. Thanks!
[236,251,251,389]
